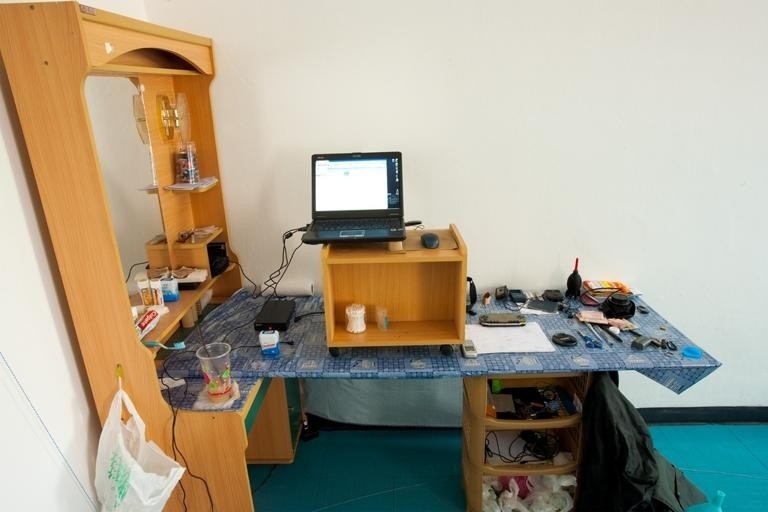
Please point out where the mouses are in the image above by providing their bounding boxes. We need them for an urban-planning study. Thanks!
[420,233,440,249]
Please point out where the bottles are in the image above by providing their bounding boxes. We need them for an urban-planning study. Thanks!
[345,302,368,335]
[174,141,200,185]
[149,278,164,307]
[258,330,280,356]
[564,256,582,298]
[374,305,388,331]
[705,489,727,512]
[491,379,503,391]
[137,278,151,308]
[160,278,178,302]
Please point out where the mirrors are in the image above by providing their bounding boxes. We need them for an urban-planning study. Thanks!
[84,74,176,304]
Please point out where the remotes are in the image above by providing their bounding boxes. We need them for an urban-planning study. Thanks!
[462,339,477,358]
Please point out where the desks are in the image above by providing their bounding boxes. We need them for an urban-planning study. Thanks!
[320,225,468,357]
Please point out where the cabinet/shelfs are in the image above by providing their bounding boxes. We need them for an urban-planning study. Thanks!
[0,1,309,511]
[157,291,721,512]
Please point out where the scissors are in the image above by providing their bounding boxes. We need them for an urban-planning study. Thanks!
[578,328,602,349]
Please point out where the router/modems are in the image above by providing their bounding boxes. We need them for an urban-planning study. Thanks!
[207,242,226,266]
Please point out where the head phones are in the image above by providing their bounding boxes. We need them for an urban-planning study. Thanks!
[521,430,559,460]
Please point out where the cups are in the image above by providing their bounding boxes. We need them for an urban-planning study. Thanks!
[195,342,232,406]
[181,306,197,329]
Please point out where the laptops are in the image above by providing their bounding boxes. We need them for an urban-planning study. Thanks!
[301,152,406,244]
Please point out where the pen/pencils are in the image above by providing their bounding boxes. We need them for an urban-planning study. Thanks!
[190,231,196,244]
[587,321,624,348]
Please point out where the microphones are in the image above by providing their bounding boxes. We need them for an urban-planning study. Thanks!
[520,459,545,464]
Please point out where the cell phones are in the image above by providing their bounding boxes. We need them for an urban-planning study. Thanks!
[545,289,565,302]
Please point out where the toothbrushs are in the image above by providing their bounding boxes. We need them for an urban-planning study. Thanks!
[142,340,186,351]
